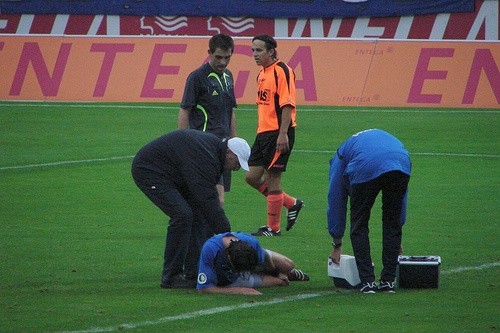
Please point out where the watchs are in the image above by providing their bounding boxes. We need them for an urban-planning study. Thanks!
[331,240,342,249]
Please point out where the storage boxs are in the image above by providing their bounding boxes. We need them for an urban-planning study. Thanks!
[327,255,375,289]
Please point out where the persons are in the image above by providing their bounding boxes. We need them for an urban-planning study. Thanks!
[131,129,252,288]
[327,127,411,293]
[177,34,238,211]
[244,34,306,236]
[196,231,310,296]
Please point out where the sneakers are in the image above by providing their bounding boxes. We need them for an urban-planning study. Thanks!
[251,225,281,236]
[288,269,309,281]
[377,280,395,293]
[286,199,304,231]
[360,281,376,293]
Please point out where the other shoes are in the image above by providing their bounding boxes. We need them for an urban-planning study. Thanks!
[160,280,194,288]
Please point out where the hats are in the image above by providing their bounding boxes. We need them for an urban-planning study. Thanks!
[252,35,277,48]
[227,137,251,171]
